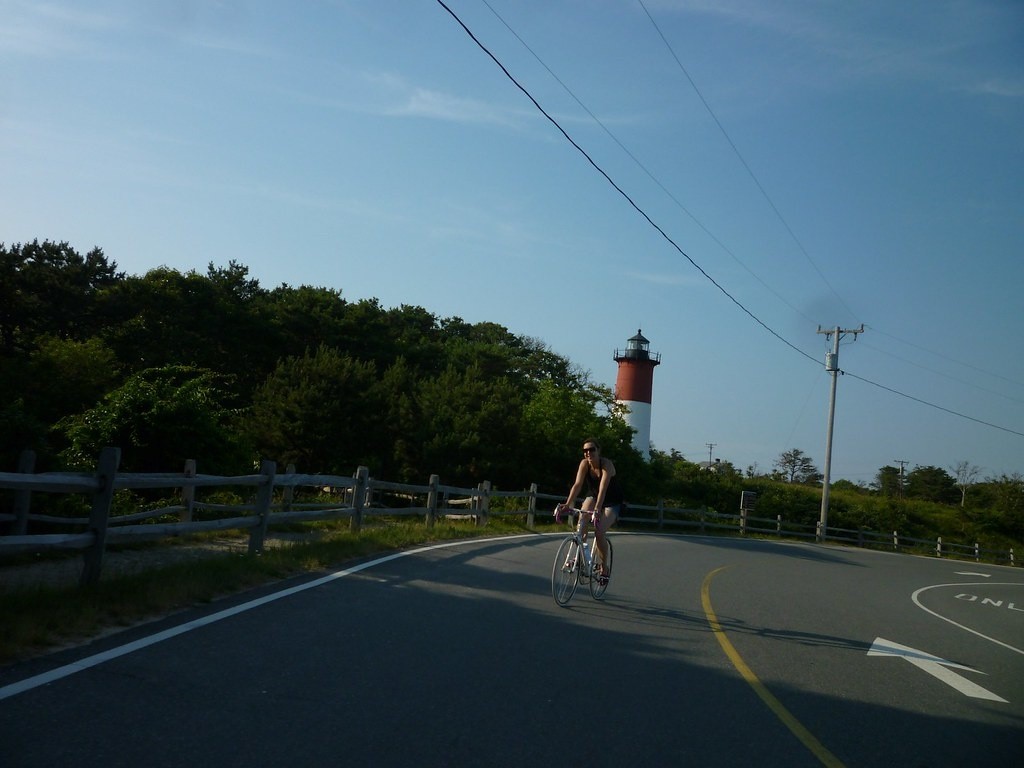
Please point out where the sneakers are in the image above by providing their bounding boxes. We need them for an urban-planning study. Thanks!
[566,558,579,569]
[599,567,610,586]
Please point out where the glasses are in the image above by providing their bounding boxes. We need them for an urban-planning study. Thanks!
[583,447,596,454]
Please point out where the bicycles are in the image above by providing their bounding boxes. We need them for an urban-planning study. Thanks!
[551,506,613,608]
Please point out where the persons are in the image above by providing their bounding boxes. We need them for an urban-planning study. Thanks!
[559,439,619,577]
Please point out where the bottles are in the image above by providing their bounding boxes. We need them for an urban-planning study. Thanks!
[583,542,591,561]
